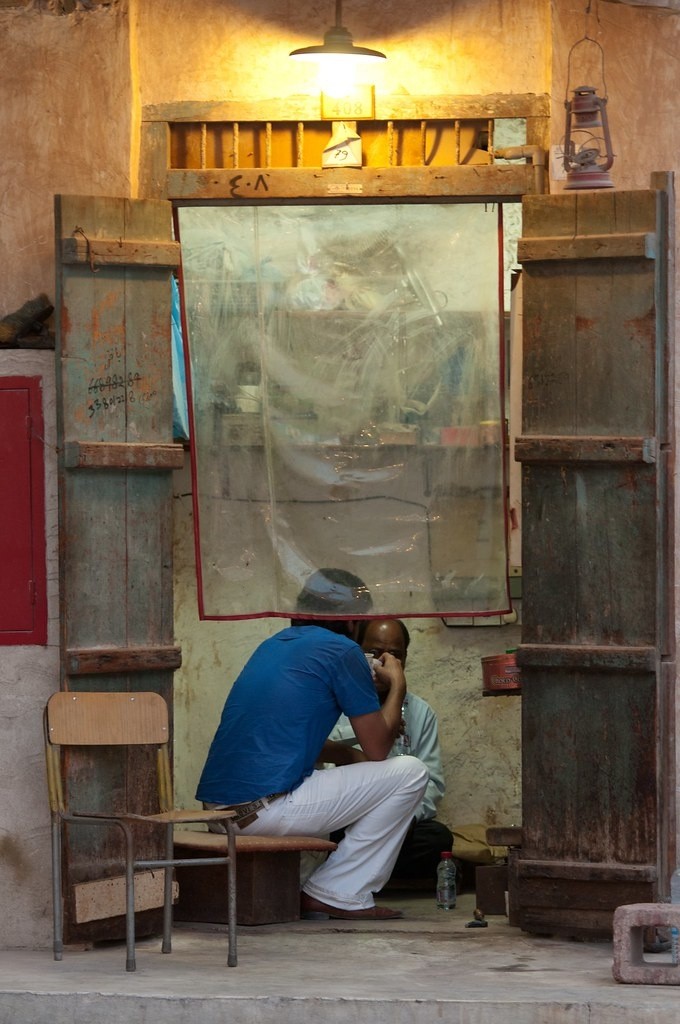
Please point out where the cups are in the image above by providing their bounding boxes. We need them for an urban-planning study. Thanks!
[363,653,375,674]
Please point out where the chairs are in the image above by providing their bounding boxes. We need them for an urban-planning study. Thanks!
[42,691,241,973]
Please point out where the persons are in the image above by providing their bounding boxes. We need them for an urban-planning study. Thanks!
[194,567,454,922]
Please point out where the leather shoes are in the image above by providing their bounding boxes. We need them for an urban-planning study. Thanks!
[301,891,405,920]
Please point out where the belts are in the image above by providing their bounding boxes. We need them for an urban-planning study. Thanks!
[231,794,279,822]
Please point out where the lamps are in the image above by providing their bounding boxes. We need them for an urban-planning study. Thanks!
[560,32,619,192]
[289,0,387,66]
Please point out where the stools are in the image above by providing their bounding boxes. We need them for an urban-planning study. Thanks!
[171,830,339,927]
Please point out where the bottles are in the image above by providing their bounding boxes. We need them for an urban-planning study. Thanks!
[392,699,410,761]
[437,852,456,910]
